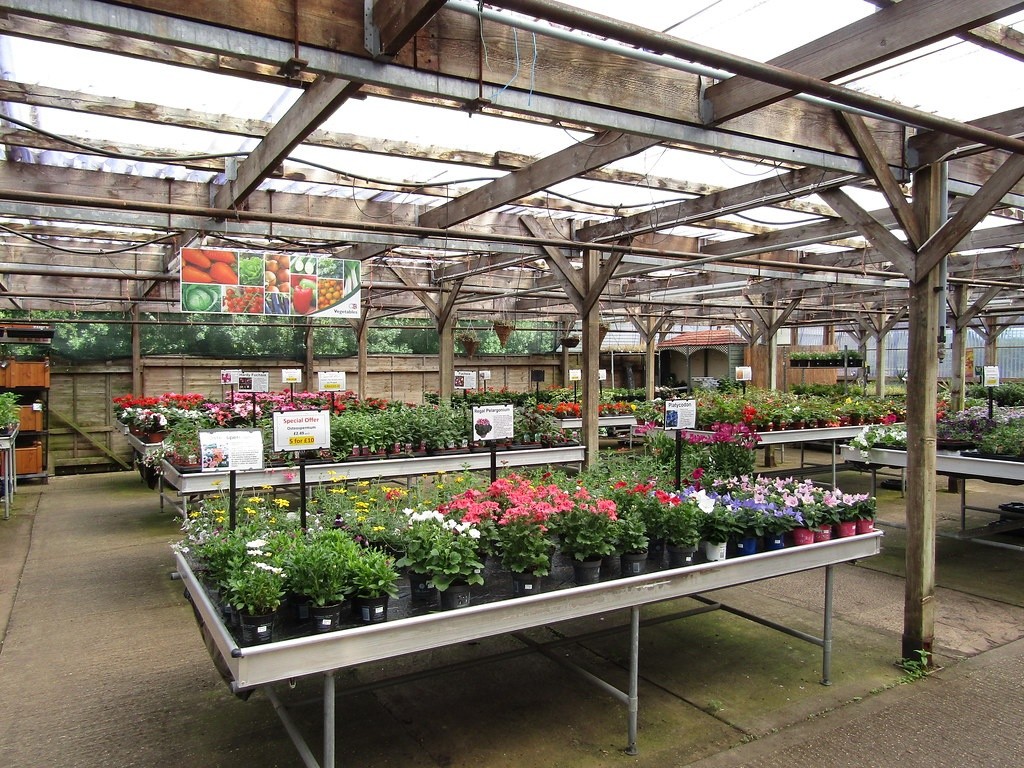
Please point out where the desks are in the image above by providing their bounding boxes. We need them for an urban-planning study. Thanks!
[114,414,1023,768]
[0,423,19,518]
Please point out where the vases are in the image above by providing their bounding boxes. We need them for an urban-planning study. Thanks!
[513,572,541,599]
[599,554,614,577]
[636,419,644,425]
[857,519,875,535]
[815,524,832,542]
[795,527,815,546]
[239,612,275,645]
[795,423,804,429]
[358,594,388,624]
[765,423,774,432]
[811,421,818,428]
[737,536,756,555]
[837,522,856,538]
[441,583,470,609]
[748,423,757,431]
[644,533,663,557]
[312,602,341,632]
[852,417,863,425]
[408,570,436,600]
[779,422,786,431]
[706,541,727,561]
[131,425,144,438]
[765,534,785,552]
[619,552,647,577]
[573,557,603,587]
[673,547,694,566]
[148,432,162,444]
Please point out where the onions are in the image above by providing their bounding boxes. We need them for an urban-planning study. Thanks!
[265,255,289,293]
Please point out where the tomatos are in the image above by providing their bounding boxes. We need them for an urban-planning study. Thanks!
[222,285,263,313]
[318,280,344,309]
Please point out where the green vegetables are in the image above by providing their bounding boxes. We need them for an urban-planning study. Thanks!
[239,256,264,285]
[316,258,343,278]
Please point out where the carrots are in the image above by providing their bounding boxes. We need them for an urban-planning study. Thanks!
[182,248,239,285]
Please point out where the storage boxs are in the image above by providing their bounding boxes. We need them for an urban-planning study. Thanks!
[0,357,49,477]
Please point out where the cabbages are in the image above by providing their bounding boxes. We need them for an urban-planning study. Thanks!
[182,284,220,312]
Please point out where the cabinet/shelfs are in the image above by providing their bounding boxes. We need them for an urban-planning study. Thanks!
[0,356,49,480]
[781,345,866,396]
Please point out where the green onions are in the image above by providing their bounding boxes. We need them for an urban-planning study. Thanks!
[343,260,360,297]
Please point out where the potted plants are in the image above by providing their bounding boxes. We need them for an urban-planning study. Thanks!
[789,350,862,367]
[557,333,582,347]
[0,391,22,437]
[492,318,514,350]
[598,316,608,350]
[457,332,480,361]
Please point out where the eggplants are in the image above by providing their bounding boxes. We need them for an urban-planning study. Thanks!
[265,293,289,314]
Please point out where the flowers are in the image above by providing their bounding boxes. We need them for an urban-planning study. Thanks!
[109,373,1024,628]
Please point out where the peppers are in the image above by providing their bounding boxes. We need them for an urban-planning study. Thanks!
[290,274,317,314]
